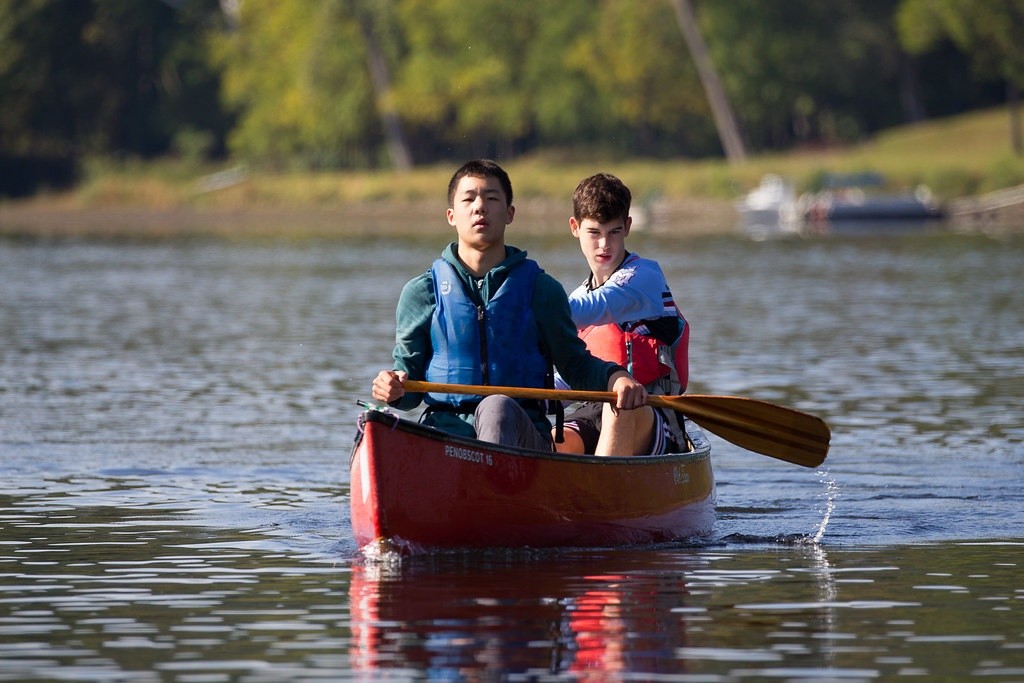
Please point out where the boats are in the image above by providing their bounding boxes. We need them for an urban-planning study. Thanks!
[349,399,716,551]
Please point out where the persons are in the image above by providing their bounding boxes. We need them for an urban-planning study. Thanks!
[550,172,688,459]
[372,159,648,452]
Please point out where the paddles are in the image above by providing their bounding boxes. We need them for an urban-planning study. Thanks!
[391,372,831,468]
